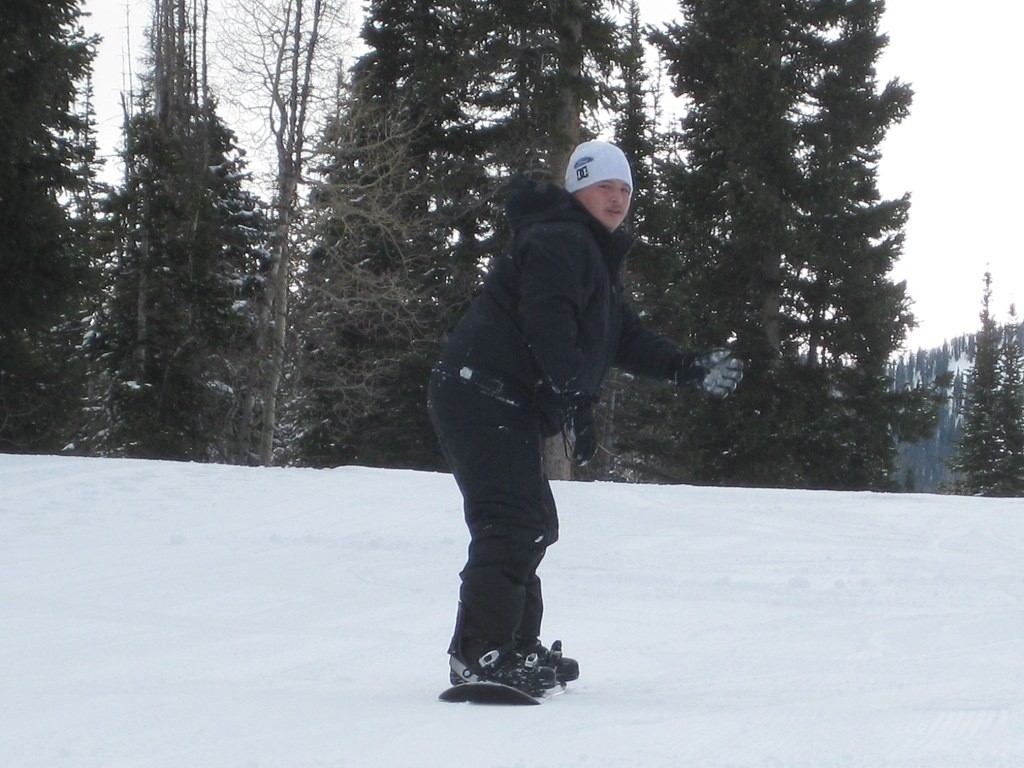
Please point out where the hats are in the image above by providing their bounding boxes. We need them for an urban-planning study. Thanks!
[566,142,633,193]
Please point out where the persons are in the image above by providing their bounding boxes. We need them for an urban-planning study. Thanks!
[427,140,744,698]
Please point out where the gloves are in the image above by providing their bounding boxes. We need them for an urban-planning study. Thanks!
[562,405,596,467]
[683,351,743,398]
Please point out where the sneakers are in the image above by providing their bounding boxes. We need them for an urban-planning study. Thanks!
[451,637,578,692]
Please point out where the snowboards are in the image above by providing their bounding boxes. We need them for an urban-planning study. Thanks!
[436,670,571,707]
